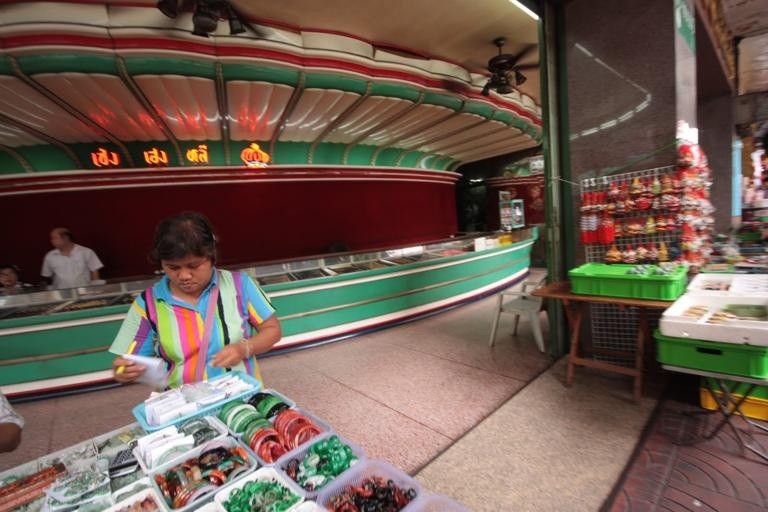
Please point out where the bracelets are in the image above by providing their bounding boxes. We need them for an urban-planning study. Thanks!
[151,390,422,512]
[239,337,254,360]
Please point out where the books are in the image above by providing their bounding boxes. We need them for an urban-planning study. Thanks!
[122,353,169,389]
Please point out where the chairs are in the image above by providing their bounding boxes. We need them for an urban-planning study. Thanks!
[489,273,551,355]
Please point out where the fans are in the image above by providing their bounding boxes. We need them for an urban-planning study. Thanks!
[445,34,542,98]
[137,0,275,47]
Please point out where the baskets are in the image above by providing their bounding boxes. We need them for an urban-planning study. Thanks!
[131,369,263,433]
[98,388,471,512]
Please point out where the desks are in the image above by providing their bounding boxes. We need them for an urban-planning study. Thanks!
[657,362,768,464]
[537,280,677,403]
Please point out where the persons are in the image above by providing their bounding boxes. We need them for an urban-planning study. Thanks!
[39,226,104,288]
[0,392,27,453]
[107,209,283,393]
[743,175,768,207]
[0,264,34,293]
